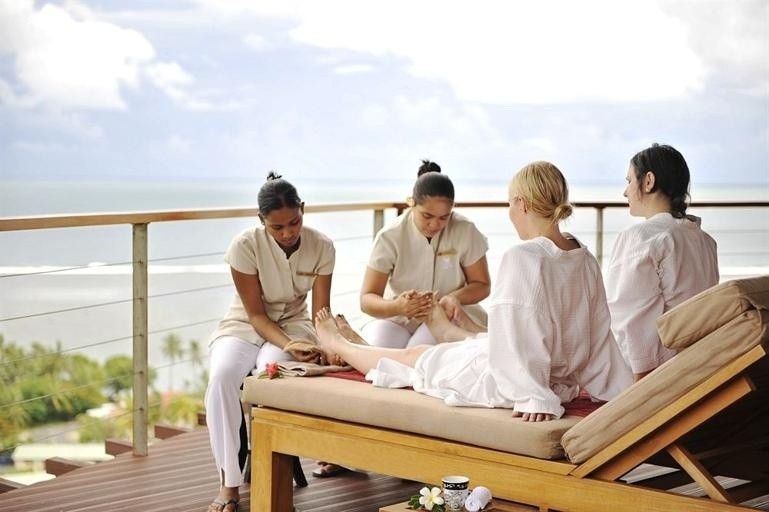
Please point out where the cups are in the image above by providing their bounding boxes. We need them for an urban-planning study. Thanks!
[441,475,471,511]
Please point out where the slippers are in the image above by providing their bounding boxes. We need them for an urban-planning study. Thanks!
[313,460,344,477]
[208,496,239,512]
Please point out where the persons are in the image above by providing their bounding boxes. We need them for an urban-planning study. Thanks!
[201,166,337,511]
[412,142,720,383]
[313,160,637,422]
[312,159,491,485]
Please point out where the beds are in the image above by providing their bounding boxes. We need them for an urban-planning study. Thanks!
[240,274,768,512]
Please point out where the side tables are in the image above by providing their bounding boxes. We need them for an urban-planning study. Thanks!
[379,488,546,512]
